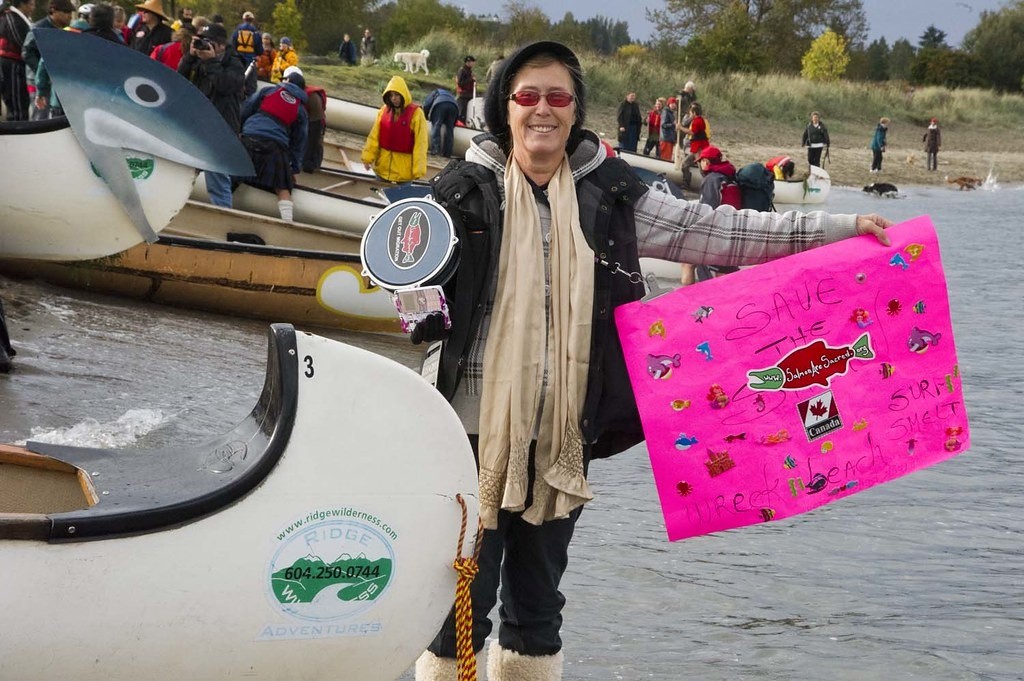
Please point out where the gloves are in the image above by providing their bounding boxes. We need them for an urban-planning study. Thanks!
[411,314,447,344]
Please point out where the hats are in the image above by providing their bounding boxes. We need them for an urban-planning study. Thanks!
[135,0,169,22]
[693,147,722,162]
[932,118,938,123]
[263,33,271,39]
[280,36,291,46]
[667,97,676,107]
[196,24,226,44]
[243,11,254,20]
[278,73,304,90]
[78,3,96,14]
[482,42,581,138]
[51,0,75,11]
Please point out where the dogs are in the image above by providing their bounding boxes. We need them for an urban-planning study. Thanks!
[394,50,429,76]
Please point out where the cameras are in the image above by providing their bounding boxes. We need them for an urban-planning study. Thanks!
[193,38,215,51]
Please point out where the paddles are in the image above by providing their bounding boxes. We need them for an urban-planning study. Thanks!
[674,94,682,170]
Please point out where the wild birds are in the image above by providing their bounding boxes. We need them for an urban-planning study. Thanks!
[946,176,983,192]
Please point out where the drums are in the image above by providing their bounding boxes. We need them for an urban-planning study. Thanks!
[360,193,460,300]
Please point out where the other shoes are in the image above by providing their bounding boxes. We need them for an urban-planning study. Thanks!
[870,168,883,173]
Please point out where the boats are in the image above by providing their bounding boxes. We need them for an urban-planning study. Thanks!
[0,72,834,336]
[0,320,488,681]
[0,24,262,261]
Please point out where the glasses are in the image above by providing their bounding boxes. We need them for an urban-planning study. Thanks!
[506,91,578,108]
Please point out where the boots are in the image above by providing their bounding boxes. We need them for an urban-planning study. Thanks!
[414,652,462,681]
[489,638,563,680]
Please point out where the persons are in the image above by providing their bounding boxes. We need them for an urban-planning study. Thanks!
[338,25,896,681]
[0,1,336,221]
[922,118,941,171]
